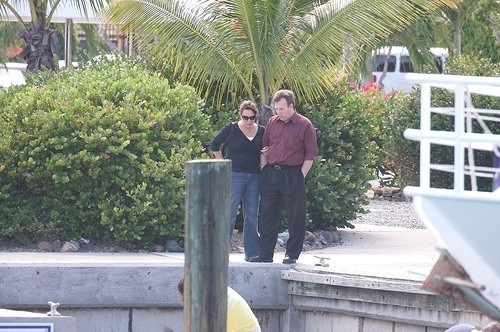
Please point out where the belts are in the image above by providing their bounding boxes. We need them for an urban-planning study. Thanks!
[272,165,292,171]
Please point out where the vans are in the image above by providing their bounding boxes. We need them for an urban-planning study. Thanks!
[364,44,450,75]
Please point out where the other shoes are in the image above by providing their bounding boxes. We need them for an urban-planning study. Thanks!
[283,255,296,264]
[249,255,274,262]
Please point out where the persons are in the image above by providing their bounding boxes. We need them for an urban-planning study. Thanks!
[177,274,261,332]
[248,88,318,264]
[210,100,267,263]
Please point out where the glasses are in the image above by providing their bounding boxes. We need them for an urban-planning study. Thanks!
[241,115,256,121]
